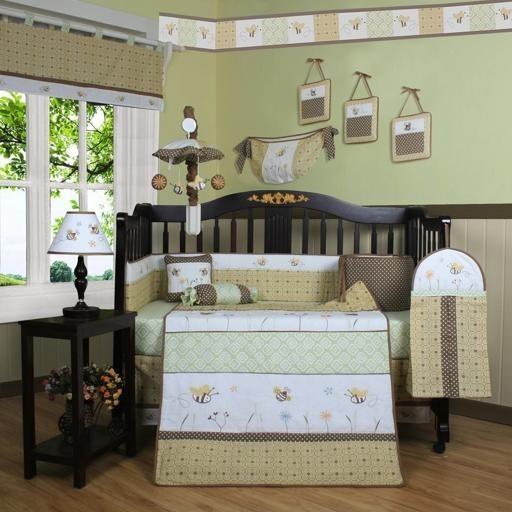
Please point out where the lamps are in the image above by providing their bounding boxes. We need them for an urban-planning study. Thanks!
[46,210,113,319]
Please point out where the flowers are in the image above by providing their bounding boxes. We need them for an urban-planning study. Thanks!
[40,362,123,413]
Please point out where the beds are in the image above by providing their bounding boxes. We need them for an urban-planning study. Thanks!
[112,189,453,456]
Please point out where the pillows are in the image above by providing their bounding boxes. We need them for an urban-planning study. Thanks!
[165,252,259,309]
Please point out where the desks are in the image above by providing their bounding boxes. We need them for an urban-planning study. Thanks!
[17,308,138,490]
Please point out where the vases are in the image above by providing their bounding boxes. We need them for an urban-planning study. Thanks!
[58,398,95,449]
